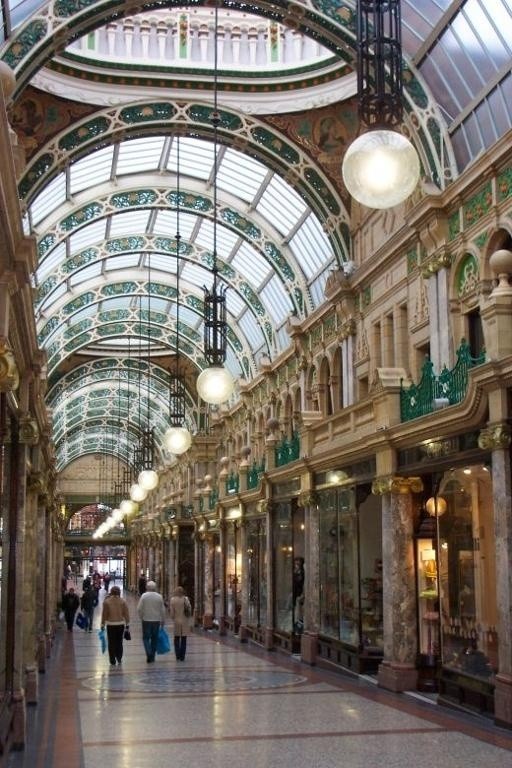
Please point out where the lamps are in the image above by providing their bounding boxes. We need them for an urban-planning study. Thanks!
[195,23,237,408]
[89,254,160,543]
[340,0,422,212]
[160,107,196,458]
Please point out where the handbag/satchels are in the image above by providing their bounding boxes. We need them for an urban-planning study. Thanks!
[157,628,170,654]
[76,613,88,629]
[183,596,192,618]
[124,629,131,641]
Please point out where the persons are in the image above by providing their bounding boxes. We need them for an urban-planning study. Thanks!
[136,581,166,663]
[138,573,146,594]
[102,586,130,665]
[170,586,192,661]
[61,569,111,633]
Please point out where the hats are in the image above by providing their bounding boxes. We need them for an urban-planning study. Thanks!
[173,586,185,595]
[145,580,157,590]
